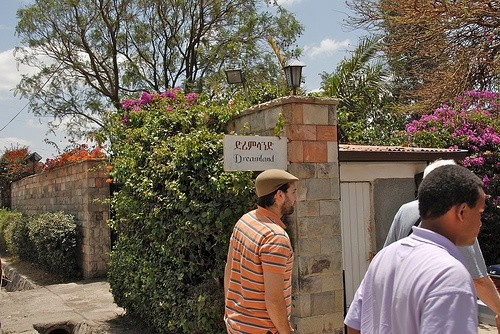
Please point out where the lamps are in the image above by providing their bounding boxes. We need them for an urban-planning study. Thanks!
[25,152,42,175]
[281,50,307,95]
[224,67,251,104]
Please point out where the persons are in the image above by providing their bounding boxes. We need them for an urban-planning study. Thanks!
[342,164,499,334]
[383,158,499,333]
[221,168,302,334]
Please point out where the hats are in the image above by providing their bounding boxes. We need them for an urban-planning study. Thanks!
[255,168,299,198]
